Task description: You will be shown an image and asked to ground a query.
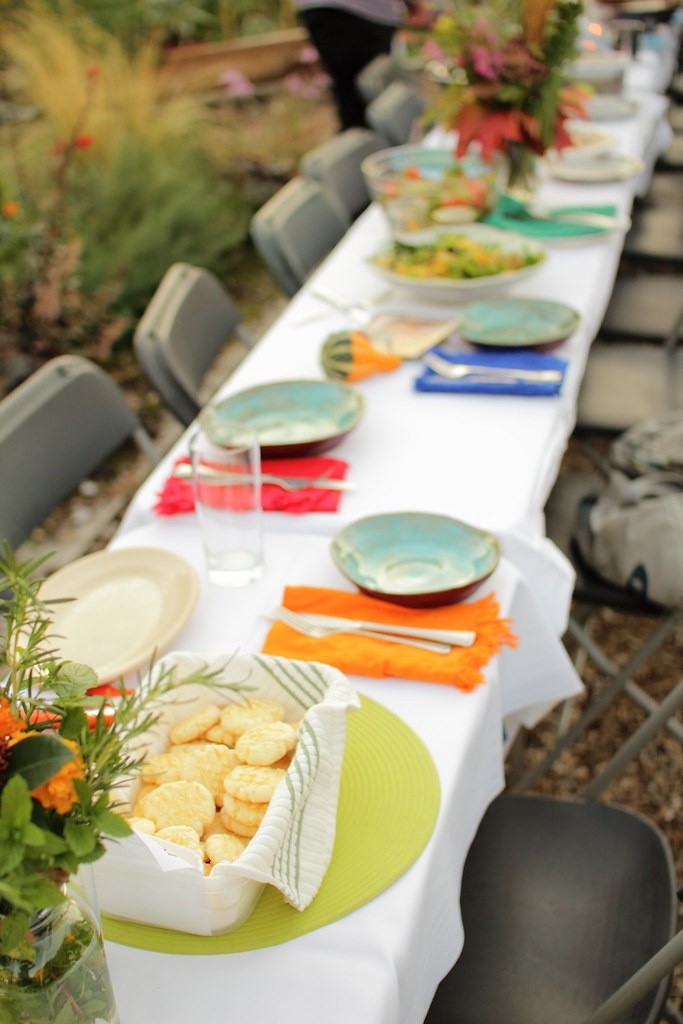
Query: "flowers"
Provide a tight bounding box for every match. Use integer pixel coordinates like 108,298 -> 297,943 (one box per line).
2,536 -> 265,989
396,1 -> 592,188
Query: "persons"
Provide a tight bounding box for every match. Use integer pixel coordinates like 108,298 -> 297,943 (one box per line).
296,1 -> 431,132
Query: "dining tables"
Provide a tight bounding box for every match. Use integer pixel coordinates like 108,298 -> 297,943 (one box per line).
1,1 -> 682,1021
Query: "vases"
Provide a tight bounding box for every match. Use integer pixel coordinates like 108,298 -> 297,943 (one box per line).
485,137 -> 550,203
1,884 -> 122,1024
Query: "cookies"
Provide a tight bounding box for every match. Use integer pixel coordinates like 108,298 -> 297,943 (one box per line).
127,694 -> 302,876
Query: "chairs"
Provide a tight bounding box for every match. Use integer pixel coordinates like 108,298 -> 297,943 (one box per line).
599,0 -> 683,347
576,302 -> 682,444
417,791 -> 683,1022
526,466 -> 683,809
302,127 -> 382,218
355,28 -> 424,149
248,175 -> 350,299
133,261 -> 256,424
1,353 -> 169,583
